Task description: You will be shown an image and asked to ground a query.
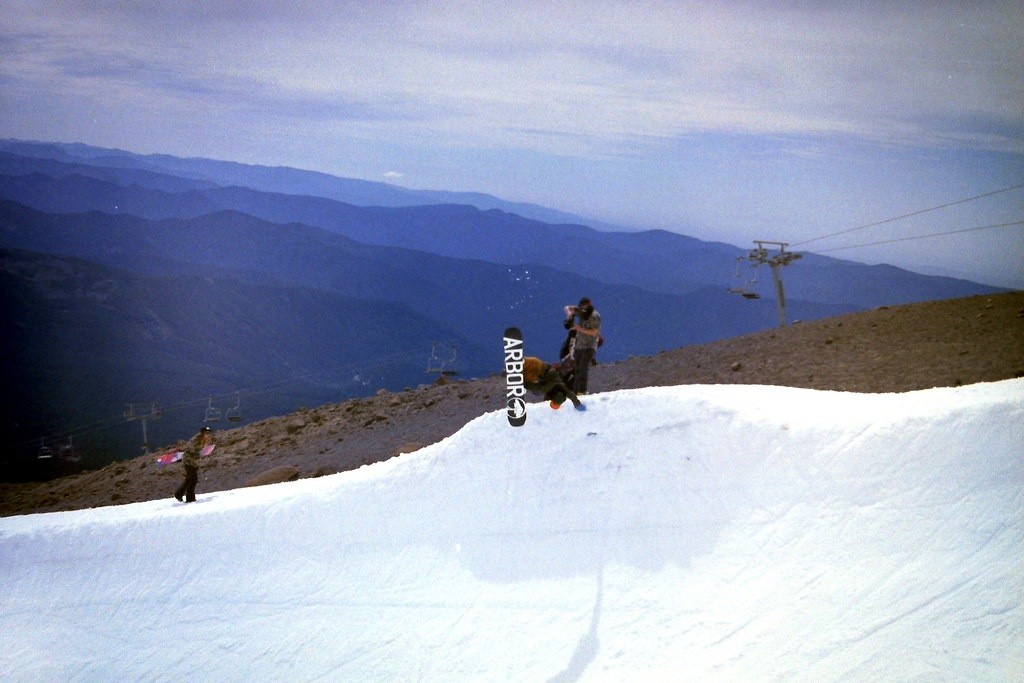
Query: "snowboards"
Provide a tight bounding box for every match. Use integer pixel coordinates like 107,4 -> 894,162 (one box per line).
503,327 -> 527,428
156,444 -> 215,465
560,313 -> 580,359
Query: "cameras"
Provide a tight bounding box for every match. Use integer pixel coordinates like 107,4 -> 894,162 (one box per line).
563,317 -> 574,329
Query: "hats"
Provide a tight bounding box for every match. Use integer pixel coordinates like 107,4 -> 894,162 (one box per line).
201,426 -> 212,432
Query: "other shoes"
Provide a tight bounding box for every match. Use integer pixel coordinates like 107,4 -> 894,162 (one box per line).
174,492 -> 185,502
186,498 -> 196,502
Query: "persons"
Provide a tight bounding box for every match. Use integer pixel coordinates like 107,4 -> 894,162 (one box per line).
501,297 -> 603,411
174,426 -> 213,503
